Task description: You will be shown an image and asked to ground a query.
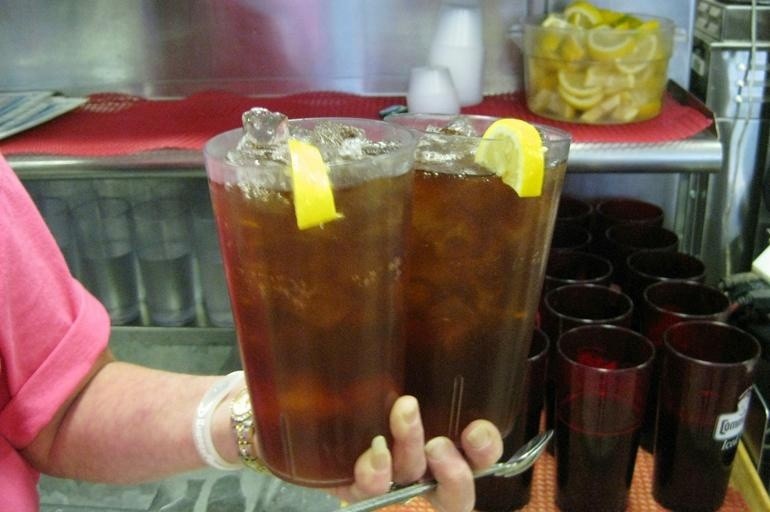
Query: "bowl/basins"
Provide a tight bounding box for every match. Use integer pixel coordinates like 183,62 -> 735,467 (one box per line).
508,10 -> 676,122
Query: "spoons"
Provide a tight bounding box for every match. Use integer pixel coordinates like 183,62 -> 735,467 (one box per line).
332,429 -> 554,510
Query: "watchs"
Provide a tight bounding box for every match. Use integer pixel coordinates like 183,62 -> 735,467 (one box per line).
231,386 -> 270,472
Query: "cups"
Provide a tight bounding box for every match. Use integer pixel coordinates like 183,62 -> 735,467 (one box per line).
621,251 -> 706,332
651,322 -> 761,511
203,115 -> 417,489
540,193 -> 683,289
37,193 -> 238,328
383,111 -> 551,452
542,284 -> 636,413
551,322 -> 652,511
641,284 -> 734,427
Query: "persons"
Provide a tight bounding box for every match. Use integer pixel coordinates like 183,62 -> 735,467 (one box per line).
0,153 -> 505,511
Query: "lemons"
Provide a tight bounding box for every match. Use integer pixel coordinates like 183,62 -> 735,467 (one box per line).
287,137 -> 345,231
528,1 -> 671,122
474,117 -> 546,198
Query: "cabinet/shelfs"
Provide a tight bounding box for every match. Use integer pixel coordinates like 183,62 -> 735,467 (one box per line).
2,76 -> 769,511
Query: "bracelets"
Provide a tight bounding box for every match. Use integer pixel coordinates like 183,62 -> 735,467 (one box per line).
193,370 -> 245,473
